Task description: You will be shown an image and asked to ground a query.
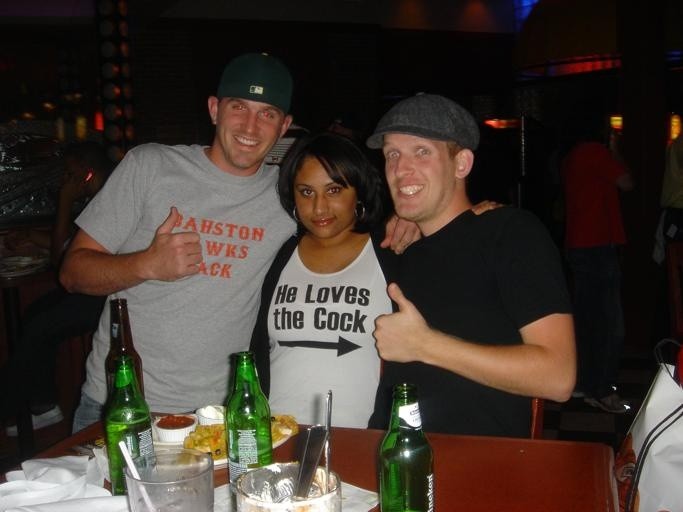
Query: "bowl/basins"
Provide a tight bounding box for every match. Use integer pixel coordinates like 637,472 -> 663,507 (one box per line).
195,405 -> 227,427
152,414 -> 198,445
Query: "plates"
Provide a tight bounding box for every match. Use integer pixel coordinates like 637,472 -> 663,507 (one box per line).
127,414 -> 291,478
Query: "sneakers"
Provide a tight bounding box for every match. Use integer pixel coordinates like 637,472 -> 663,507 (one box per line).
572,387 -> 631,416
5,403 -> 63,438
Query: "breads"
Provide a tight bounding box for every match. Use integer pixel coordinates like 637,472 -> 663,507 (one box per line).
174,412 -> 299,466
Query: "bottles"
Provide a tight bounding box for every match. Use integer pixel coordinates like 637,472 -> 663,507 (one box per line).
105,298 -> 145,405
101,352 -> 155,496
225,351 -> 273,498
378,380 -> 434,512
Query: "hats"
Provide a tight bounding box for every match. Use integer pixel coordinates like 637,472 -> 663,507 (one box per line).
217,52 -> 294,116
365,92 -> 481,150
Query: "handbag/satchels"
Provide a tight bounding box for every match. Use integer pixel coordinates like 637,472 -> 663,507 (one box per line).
610,339 -> 683,512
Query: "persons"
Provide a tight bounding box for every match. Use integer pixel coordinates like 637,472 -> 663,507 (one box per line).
0,139 -> 124,439
51,44 -> 426,440
252,123 -> 510,433
366,90 -> 580,443
658,132 -> 682,342
550,118 -> 635,415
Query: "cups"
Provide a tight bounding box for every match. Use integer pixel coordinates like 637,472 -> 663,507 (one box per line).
233,461 -> 342,512
121,447 -> 215,512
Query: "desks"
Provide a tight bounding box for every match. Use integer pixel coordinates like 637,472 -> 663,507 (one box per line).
0,411 -> 618,512
